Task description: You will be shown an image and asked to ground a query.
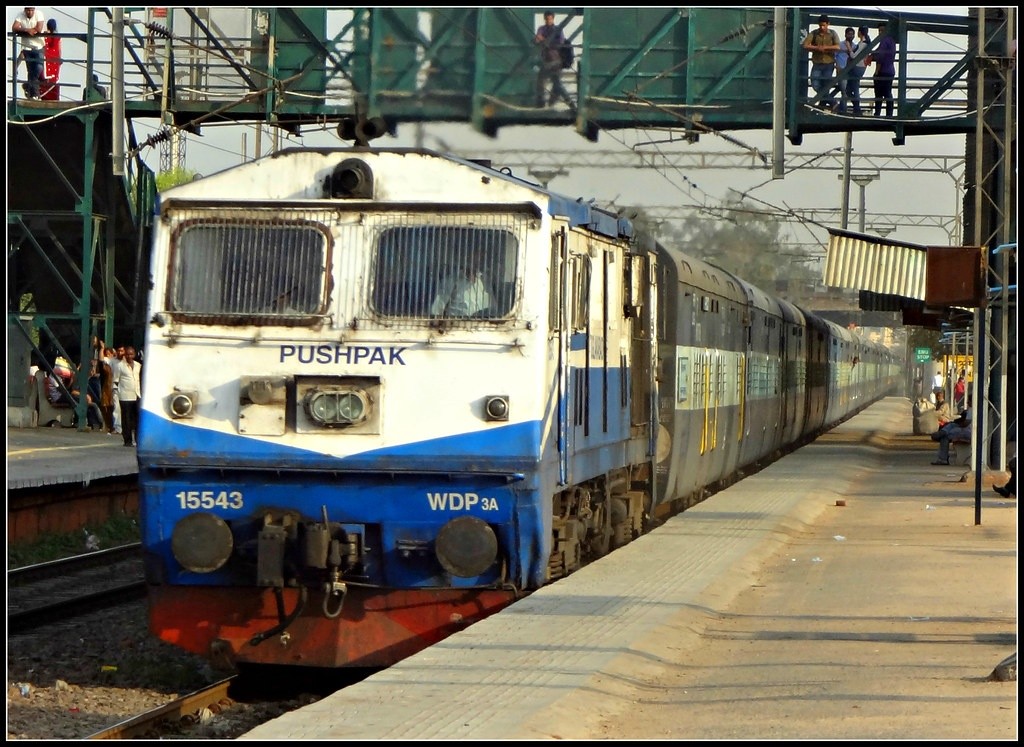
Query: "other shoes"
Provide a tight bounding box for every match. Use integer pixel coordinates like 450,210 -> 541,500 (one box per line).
931,432 -> 941,442
122,442 -> 135,447
992,484 -> 1010,498
930,460 -> 950,465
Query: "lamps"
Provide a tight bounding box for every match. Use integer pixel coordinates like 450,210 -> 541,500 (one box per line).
305,385 -> 374,427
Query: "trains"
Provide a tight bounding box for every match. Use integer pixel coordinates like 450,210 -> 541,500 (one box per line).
132,132 -> 911,669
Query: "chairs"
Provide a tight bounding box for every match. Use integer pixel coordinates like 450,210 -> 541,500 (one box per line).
35,370 -> 74,427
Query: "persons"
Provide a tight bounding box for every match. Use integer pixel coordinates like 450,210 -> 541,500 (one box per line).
532,11 -> 575,108
931,371 -> 1018,498
11,7 -> 107,102
29,335 -> 143,447
428,257 -> 499,318
261,282 -> 302,314
802,15 -> 896,117
